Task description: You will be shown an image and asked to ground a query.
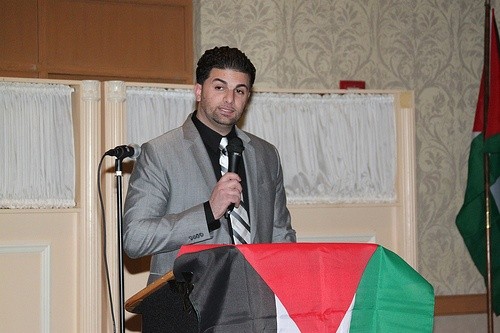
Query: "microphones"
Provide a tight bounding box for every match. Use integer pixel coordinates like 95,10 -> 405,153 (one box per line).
105,142 -> 142,159
227,137 -> 244,213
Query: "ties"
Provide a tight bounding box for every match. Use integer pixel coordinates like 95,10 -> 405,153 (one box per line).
218,137 -> 251,245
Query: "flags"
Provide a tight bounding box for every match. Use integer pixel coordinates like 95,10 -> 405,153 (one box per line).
172,243 -> 435,333
455,0 -> 500,320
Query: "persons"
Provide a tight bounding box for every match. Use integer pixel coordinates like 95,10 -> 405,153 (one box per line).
121,44 -> 297,333
